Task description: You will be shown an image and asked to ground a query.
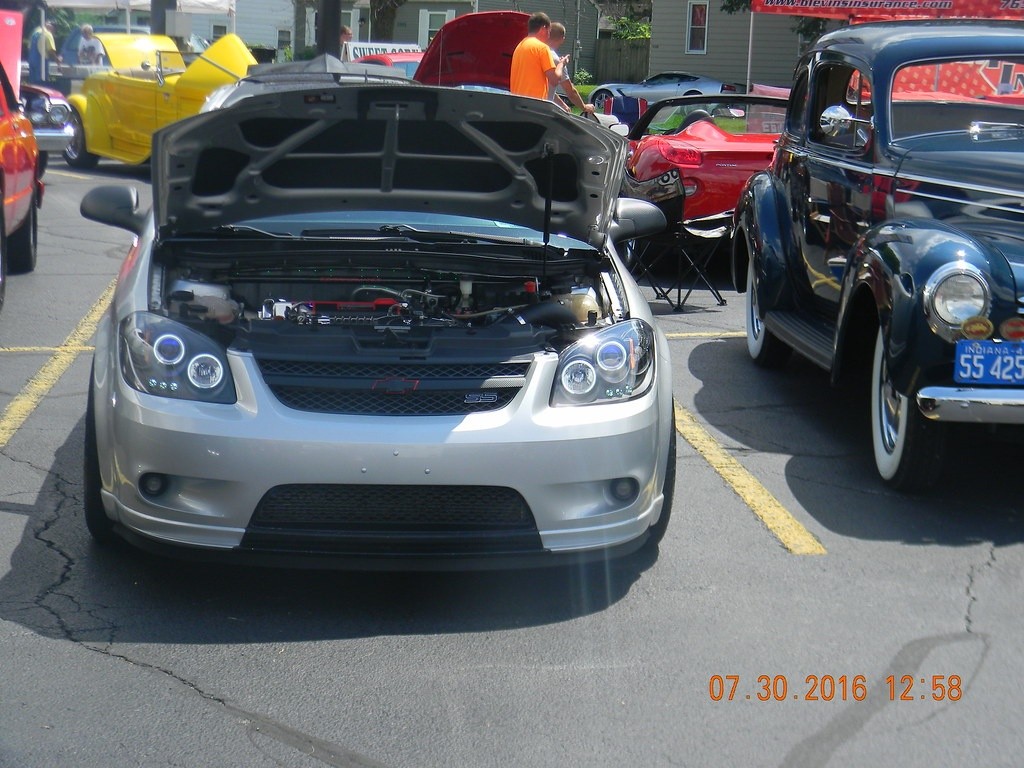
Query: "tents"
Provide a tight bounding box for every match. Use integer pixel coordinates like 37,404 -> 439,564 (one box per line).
744,0 -> 1024,133
36,0 -> 236,81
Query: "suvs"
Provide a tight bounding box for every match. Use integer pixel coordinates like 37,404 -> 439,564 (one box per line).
734,27 -> 1022,492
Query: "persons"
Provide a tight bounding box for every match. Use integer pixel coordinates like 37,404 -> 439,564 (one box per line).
27,21 -> 63,86
548,22 -> 595,115
329,26 -> 353,60
77,24 -> 105,66
510,12 -> 570,100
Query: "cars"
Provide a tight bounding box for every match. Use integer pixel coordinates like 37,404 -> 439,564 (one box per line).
82,75 -> 678,571
351,10 -> 532,92
0,11 -> 48,308
588,70 -> 736,128
19,85 -> 75,182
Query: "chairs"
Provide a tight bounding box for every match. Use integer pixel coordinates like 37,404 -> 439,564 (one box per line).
675,110 -> 718,136
622,171 -> 734,311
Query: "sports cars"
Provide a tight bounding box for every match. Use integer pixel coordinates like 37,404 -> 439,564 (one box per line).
65,31 -> 262,178
605,92 -> 802,259
